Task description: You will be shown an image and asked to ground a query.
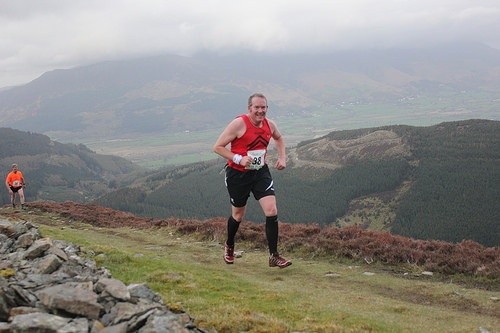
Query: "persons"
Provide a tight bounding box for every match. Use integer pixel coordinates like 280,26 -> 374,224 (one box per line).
214,94 -> 292,268
6,164 -> 27,210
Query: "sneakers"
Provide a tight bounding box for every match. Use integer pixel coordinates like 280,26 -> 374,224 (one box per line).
269,252 -> 292,268
224,240 -> 235,263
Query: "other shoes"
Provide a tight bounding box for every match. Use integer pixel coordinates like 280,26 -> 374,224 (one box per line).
21,204 -> 24,209
12,204 -> 17,209
